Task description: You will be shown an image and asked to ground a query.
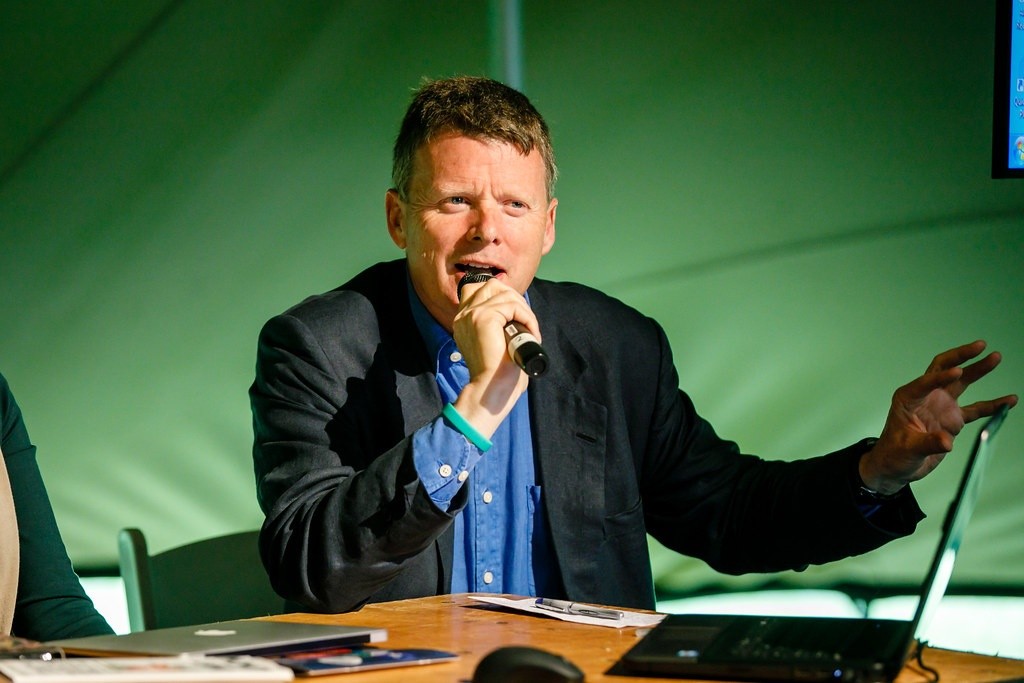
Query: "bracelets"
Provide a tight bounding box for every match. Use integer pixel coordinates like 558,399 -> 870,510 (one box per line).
857,441 -> 903,506
442,401 -> 493,453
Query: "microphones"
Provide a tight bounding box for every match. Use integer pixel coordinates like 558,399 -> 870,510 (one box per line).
457,268 -> 550,378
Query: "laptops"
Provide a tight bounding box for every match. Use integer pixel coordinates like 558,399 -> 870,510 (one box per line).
603,401 -> 1010,683
41,619 -> 389,660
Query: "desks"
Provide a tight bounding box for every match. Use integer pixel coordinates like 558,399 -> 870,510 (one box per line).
0,593 -> 1024,683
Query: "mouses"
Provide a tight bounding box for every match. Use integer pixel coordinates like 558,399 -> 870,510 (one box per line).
472,646 -> 583,683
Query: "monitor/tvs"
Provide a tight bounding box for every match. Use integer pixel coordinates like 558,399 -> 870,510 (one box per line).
990,0 -> 1024,179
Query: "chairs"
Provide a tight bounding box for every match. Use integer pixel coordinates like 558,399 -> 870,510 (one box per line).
117,528 -> 285,634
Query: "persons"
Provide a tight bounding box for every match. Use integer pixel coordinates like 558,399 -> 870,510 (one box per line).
0,373 -> 118,643
248,76 -> 1019,616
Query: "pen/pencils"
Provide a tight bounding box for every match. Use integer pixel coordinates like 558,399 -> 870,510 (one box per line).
534,596 -> 624,621
